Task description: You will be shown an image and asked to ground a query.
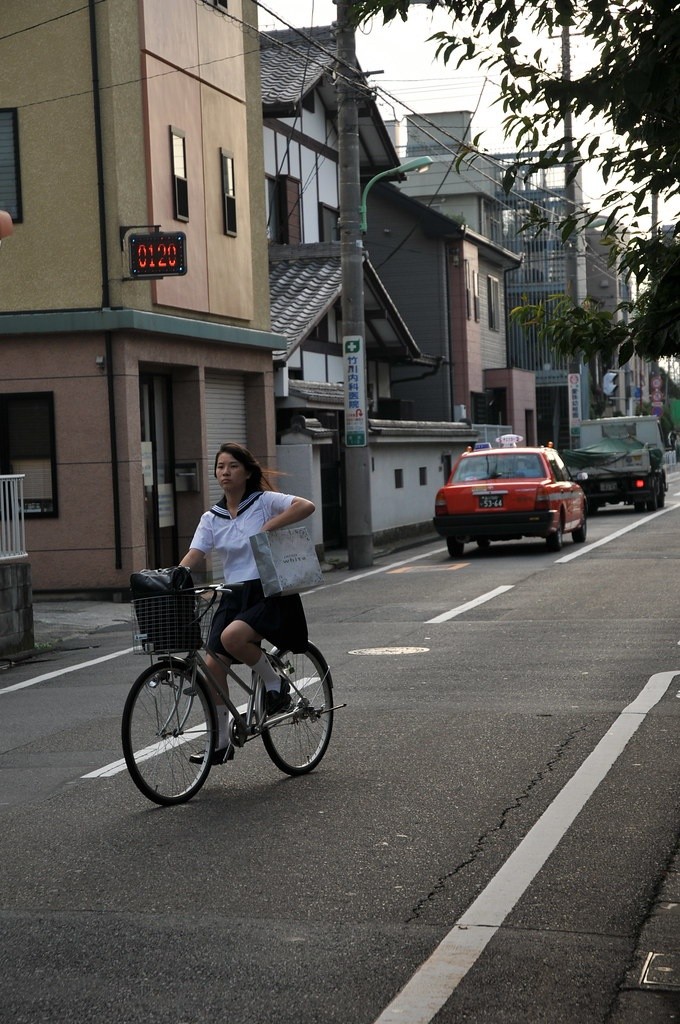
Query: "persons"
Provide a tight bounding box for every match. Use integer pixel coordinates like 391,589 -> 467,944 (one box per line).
178,441 -> 316,766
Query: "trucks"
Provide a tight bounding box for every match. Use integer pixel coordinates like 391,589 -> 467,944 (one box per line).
558,412 -> 676,515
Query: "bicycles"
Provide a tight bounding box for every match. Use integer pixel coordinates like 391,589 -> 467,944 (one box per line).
120,580 -> 349,807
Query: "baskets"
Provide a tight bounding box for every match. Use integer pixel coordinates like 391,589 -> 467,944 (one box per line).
131,594 -> 214,655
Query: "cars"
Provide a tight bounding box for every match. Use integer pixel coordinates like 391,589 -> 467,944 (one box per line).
433,434 -> 589,557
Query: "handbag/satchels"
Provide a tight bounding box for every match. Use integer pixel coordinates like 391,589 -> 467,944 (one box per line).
249,496 -> 324,597
129,566 -> 203,650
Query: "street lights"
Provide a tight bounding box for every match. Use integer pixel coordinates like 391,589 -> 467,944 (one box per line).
336,154 -> 435,570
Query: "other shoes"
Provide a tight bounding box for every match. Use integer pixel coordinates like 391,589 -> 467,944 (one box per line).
265,674 -> 286,715
190,746 -> 236,764
